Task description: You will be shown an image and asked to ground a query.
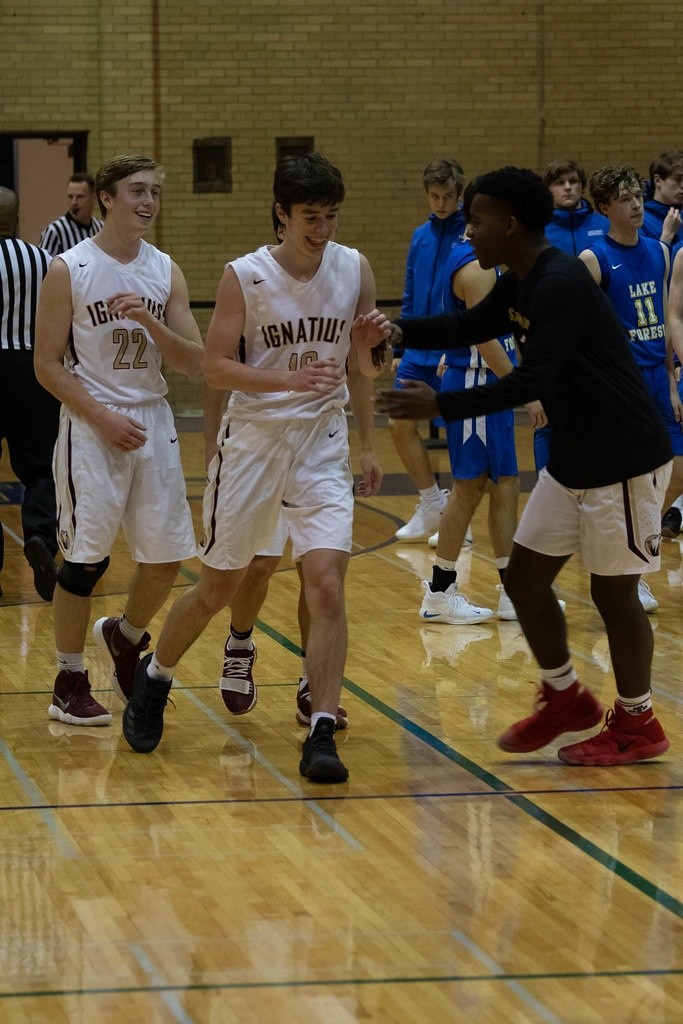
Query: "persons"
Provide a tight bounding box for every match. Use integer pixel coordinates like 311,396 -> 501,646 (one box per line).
220,198 -> 349,728
371,165 -> 673,767
39,173 -> 104,257
33,152 -> 204,728
576,165 -> 683,612
639,146 -> 683,245
419,183 -> 567,624
532,158 -> 610,479
389,157 -> 471,547
121,151 -> 392,785
661,245 -> 682,545
0,187 -> 57,602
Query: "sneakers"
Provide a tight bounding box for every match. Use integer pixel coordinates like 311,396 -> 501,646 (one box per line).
397,543 -> 473,582
496,676 -> 604,753
419,580 -> 492,624
660,507 -> 682,539
417,624 -> 494,666
93,615 -> 152,704
222,734 -> 257,805
219,634 -> 256,713
557,697 -> 670,765
300,717 -> 349,784
428,520 -> 473,546
396,488 -> 450,540
496,584 -> 567,621
49,720 -> 123,774
23,536 -> 59,602
48,669 -> 113,726
495,625 -> 535,665
637,579 -> 659,612
295,677 -> 348,729
121,652 -> 172,753
